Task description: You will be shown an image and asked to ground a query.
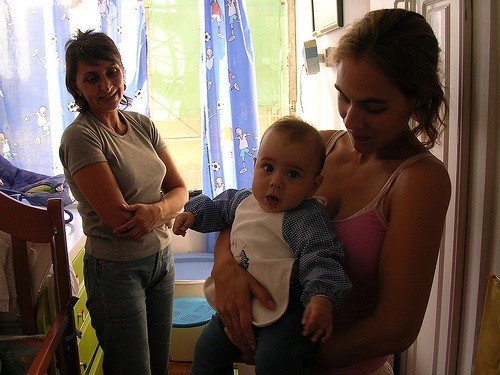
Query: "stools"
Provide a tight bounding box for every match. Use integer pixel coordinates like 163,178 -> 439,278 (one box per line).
169,297 -> 217,362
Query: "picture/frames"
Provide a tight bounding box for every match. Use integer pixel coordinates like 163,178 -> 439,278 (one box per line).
310,0 -> 343,37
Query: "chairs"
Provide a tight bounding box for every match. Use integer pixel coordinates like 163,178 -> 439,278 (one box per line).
0,190 -> 79,375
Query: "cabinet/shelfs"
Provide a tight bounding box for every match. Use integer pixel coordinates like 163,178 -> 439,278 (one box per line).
0,247 -> 103,375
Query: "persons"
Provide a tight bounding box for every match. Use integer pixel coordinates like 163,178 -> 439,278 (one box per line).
59,28 -> 189,375
173,115 -> 352,375
210,8 -> 452,375
